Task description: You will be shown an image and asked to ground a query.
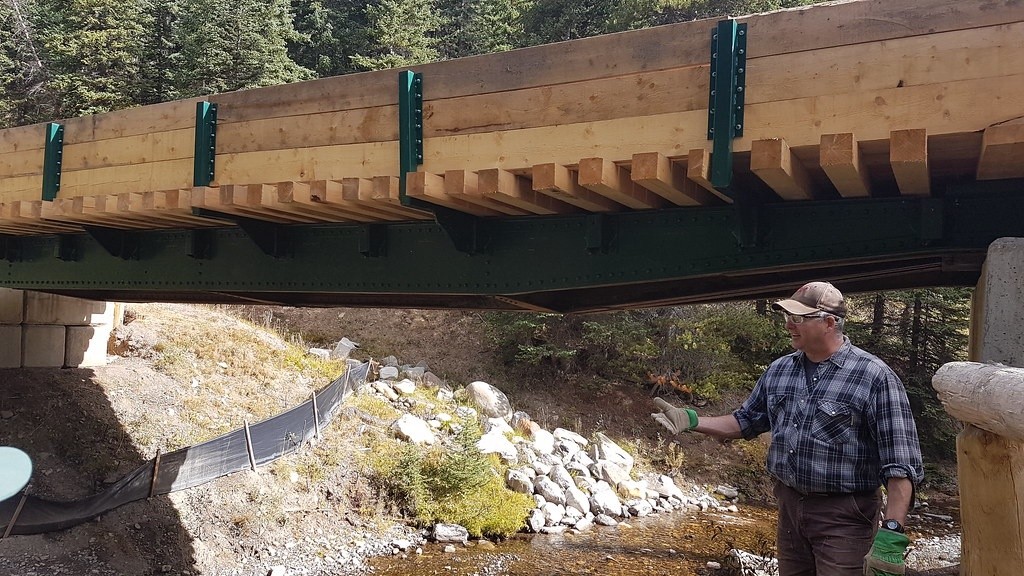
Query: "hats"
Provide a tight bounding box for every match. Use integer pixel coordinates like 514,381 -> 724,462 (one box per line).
772,281 -> 846,319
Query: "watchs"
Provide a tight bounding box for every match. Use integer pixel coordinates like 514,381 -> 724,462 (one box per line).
881,518 -> 904,533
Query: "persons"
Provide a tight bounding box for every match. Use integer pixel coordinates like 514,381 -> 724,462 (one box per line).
652,280 -> 924,576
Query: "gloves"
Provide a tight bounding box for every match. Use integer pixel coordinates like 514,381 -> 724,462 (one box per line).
650,397 -> 699,435
863,528 -> 911,576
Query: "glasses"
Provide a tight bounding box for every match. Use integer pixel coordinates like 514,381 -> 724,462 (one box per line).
783,311 -> 839,324
